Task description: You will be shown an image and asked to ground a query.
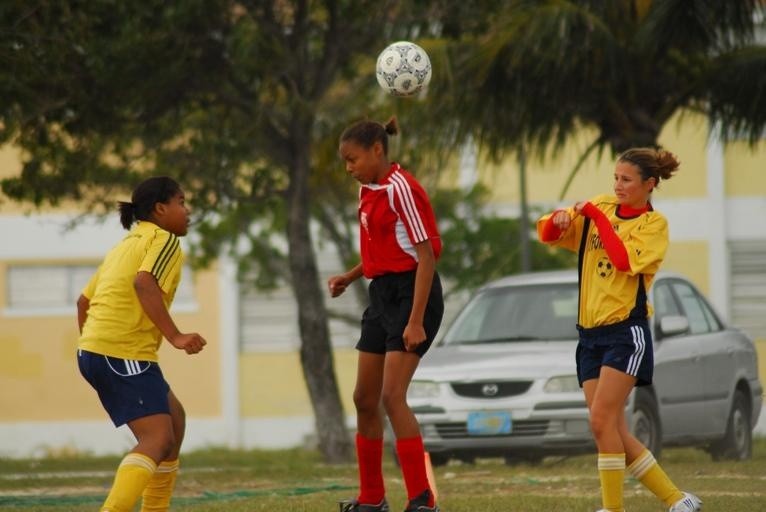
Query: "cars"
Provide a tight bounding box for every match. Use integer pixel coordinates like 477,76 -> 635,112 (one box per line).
404,271 -> 765,460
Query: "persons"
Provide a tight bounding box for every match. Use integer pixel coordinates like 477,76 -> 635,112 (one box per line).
76,176 -> 207,512
327,117 -> 444,512
537,146 -> 702,512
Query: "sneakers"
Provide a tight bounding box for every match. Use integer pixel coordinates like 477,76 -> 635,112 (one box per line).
333,493 -> 391,512
668,488 -> 704,512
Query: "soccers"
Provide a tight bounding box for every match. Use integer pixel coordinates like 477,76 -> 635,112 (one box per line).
375,40 -> 431,96
